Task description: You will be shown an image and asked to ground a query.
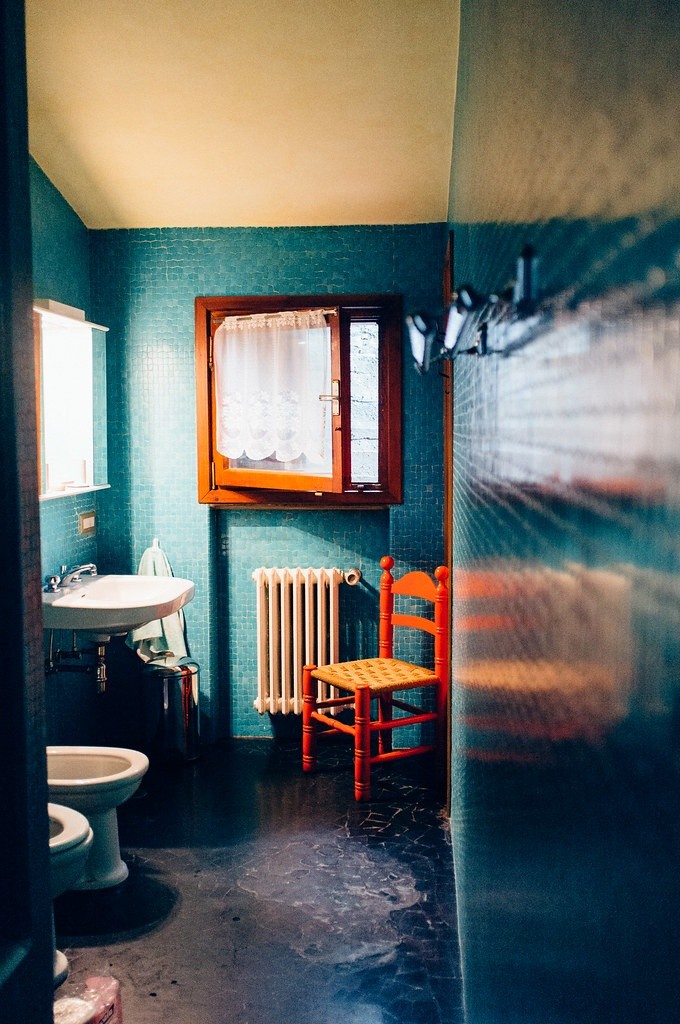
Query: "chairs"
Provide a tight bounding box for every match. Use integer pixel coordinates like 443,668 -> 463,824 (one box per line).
302,555 -> 452,800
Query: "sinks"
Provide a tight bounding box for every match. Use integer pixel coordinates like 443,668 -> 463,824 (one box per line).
42,574 -> 195,629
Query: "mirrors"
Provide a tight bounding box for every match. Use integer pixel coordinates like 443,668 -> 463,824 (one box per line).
33,297 -> 112,501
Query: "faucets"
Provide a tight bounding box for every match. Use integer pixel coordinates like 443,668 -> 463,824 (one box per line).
44,563 -> 98,593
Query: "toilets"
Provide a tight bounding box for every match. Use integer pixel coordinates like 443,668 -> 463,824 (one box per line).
47,803 -> 94,987
47,745 -> 149,892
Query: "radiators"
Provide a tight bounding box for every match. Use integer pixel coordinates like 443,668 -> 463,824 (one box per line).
251,569 -> 356,714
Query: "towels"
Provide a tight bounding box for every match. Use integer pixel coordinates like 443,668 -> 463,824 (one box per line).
125,536 -> 189,662
